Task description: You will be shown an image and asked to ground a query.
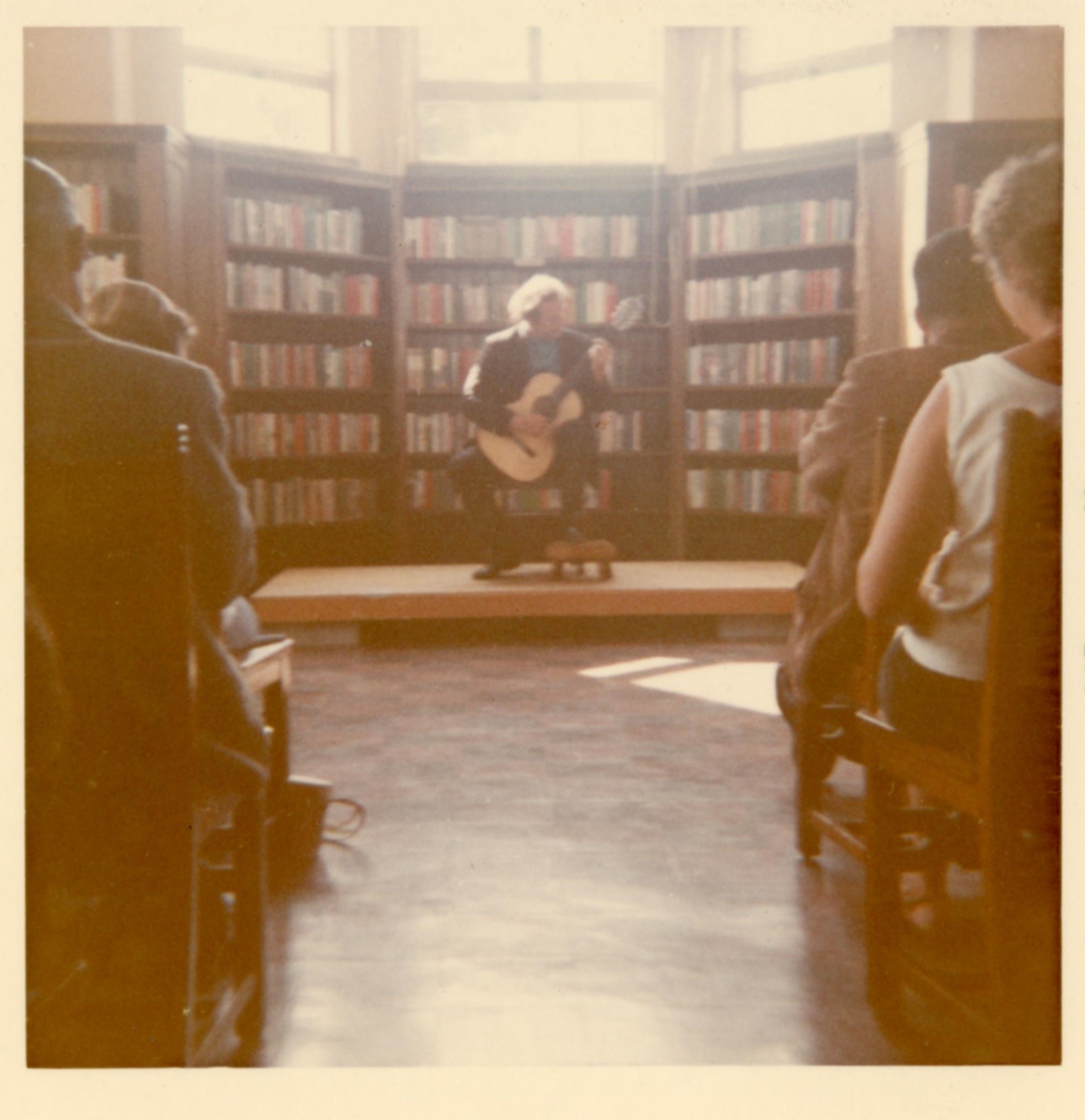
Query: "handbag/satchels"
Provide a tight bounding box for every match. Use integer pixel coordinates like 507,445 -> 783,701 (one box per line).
282,773 -> 364,860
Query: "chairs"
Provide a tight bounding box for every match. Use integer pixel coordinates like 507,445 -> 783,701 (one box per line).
795,406 -> 1061,1064
29,426 -> 295,1067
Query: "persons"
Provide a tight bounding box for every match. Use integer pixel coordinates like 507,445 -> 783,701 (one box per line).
23,152 -> 276,992
81,277 -> 260,653
786,224 -> 1028,712
855,138 -> 1064,763
445,271 -> 617,581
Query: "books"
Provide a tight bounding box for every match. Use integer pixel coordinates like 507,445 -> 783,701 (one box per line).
685,469 -> 800,516
226,196 -> 364,255
407,466 -> 610,512
685,266 -> 840,321
70,247 -> 126,326
686,335 -> 839,387
225,339 -> 374,388
404,409 -> 642,452
68,183 -> 113,232
225,260 -> 380,316
684,408 -> 817,453
401,213 -> 640,259
406,347 -> 632,392
247,477 -> 363,526
231,412 -> 381,456
686,196 -> 852,259
409,280 -> 618,322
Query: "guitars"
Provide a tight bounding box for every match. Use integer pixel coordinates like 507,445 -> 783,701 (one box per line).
475,293 -> 653,484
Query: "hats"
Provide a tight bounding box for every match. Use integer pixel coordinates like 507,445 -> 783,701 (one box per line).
914,225 -> 990,308
91,281 -> 174,332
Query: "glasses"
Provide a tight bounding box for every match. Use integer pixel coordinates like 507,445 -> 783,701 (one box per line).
550,311 -> 563,320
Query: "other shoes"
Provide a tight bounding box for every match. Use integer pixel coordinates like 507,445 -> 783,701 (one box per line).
561,526 -> 584,545
472,565 -> 501,578
897,854 -> 945,936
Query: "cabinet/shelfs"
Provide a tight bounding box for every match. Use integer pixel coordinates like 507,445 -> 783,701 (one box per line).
177,137 -> 402,571
675,131 -> 897,559
898,116 -> 1068,354
391,157 -> 674,568
24,120 -> 185,314
